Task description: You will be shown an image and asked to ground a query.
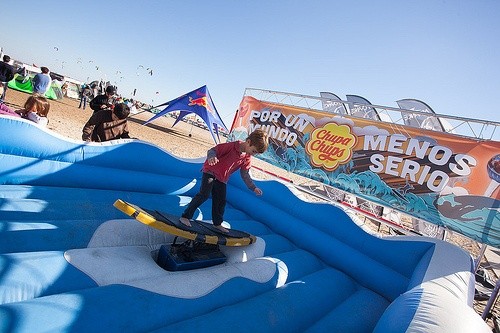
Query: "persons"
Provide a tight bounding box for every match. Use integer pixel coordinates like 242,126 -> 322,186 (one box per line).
179,128 -> 270,233
0,55 -> 131,142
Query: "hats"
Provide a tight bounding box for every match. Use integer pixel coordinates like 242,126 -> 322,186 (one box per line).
106,85 -> 116,92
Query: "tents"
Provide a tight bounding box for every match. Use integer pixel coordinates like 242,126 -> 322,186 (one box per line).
131,84 -> 230,145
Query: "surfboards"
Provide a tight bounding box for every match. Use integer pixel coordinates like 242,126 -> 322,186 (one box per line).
112,196 -> 256,248
